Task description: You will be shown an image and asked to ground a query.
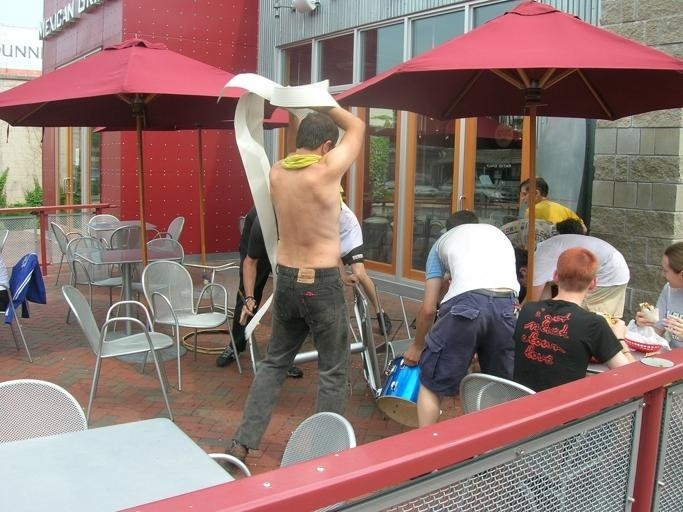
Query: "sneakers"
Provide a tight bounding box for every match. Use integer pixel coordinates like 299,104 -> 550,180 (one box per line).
225,439 -> 248,468
288,366 -> 303,378
216,345 -> 241,367
376,312 -> 392,335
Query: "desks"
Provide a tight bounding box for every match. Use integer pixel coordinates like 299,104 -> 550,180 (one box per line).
89,220 -> 155,287
1,418 -> 235,511
247,283 -> 380,402
74,250 -> 187,362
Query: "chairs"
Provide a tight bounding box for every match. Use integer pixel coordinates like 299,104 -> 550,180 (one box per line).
346,292 -> 413,382
208,412 -> 356,482
61,284 -> 177,423
114,238 -> 184,331
183,261 -> 240,311
149,216 -> 185,240
0,253 -> 38,363
88,214 -> 120,248
1,379 -> 87,444
0,230 -> 9,249
457,374 -> 608,512
67,236 -> 124,331
141,260 -> 242,391
50,222 -> 88,286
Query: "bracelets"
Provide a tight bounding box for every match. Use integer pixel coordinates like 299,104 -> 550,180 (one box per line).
617,338 -> 625,341
245,296 -> 255,303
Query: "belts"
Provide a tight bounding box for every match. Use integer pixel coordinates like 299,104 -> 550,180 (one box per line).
473,288 -> 516,299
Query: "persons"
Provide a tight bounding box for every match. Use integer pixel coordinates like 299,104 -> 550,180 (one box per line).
220,105 -> 367,476
0,253 -> 12,311
515,233 -> 631,320
216,207 -> 304,378
635,242 -> 683,348
513,247 -> 636,392
403,210 -> 522,428
338,201 -> 392,335
519,177 -> 588,234
499,217 -> 585,250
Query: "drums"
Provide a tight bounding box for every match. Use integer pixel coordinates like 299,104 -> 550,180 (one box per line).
375,352 -> 421,427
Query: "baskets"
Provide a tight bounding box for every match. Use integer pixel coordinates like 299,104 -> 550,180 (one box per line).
624,337 -> 663,353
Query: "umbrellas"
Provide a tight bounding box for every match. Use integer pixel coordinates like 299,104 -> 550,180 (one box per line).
370,111 -> 522,187
331,0 -> 683,302
92,105 -> 291,265
0,39 -> 268,268
